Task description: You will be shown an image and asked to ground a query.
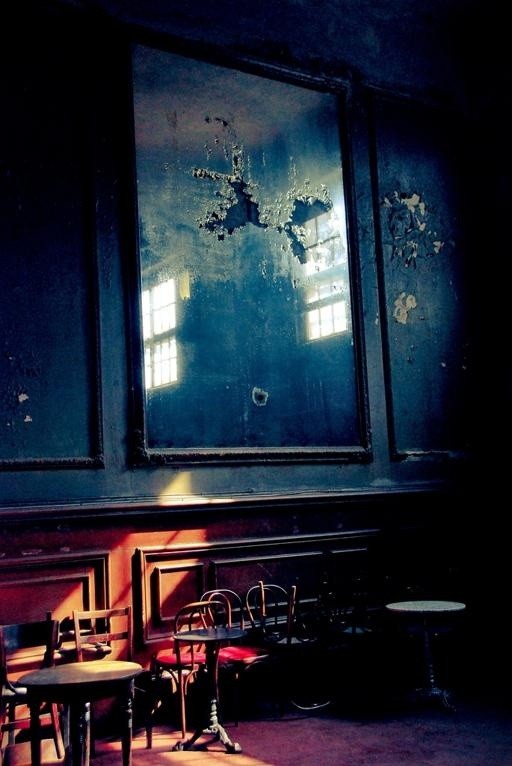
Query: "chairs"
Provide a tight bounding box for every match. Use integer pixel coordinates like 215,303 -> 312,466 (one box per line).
0,618 -> 66,766
158,599 -> 224,738
199,586 -> 271,726
73,604 -> 133,757
246,584 -> 319,715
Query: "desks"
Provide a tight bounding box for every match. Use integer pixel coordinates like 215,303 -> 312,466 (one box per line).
385,601 -> 467,706
172,631 -> 249,754
16,659 -> 142,766
